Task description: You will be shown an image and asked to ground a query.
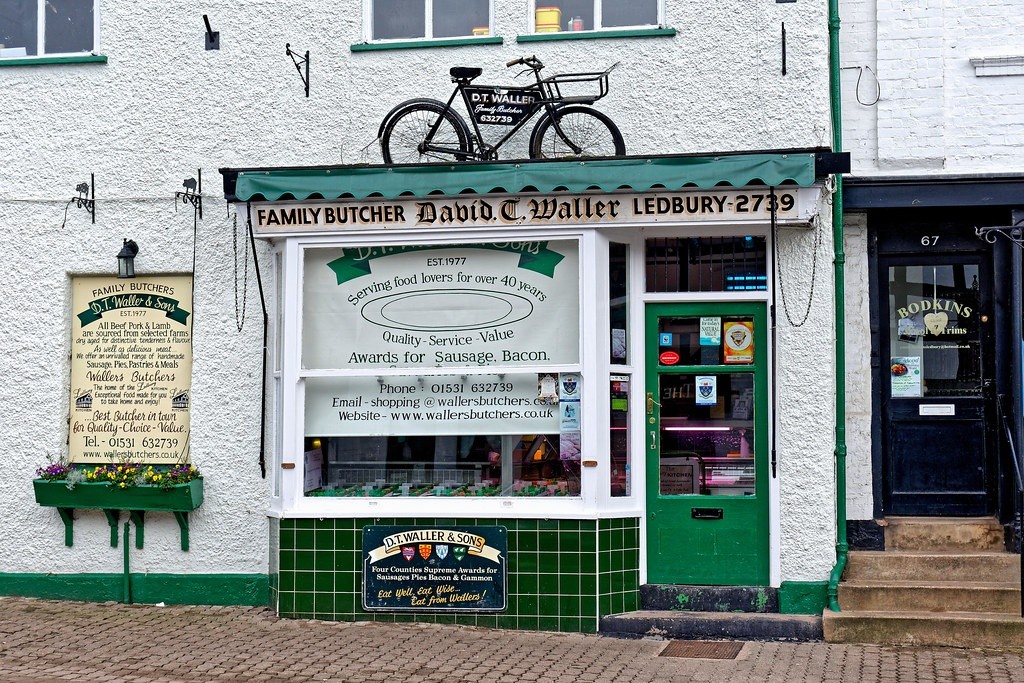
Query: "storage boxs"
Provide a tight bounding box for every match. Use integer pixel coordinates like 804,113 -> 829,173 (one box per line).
535,26 -> 563,32
472,26 -> 489,36
535,6 -> 563,26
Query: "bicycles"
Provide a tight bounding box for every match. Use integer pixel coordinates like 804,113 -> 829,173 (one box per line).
381,54 -> 626,167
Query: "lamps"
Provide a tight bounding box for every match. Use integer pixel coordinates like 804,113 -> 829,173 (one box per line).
116,238 -> 140,279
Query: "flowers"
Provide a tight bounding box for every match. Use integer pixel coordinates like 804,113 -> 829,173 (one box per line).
35,451 -> 204,492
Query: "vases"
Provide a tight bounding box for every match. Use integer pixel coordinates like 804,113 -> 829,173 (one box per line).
32,465 -> 204,552
